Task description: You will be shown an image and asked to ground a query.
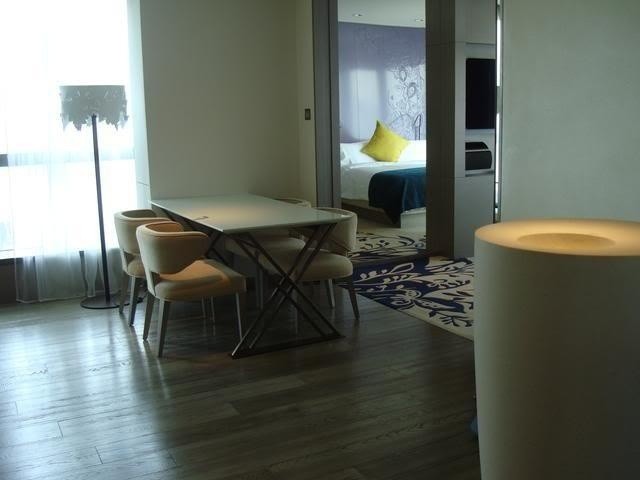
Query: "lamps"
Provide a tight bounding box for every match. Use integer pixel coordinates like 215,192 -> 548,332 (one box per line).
58,83 -> 144,312
469,216 -> 639,479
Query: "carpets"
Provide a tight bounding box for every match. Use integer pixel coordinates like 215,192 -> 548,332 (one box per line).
327,213 -> 474,342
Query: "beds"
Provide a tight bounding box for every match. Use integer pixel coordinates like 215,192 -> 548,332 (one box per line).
339,139 -> 427,230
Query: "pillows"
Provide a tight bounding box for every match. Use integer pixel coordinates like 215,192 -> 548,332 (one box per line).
359,119 -> 410,164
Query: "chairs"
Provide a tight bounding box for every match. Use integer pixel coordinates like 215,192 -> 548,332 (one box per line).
256,202 -> 362,337
223,197 -> 317,312
135,216 -> 247,359
113,208 -> 211,328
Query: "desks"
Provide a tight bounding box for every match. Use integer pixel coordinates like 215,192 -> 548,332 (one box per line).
150,191 -> 354,360
